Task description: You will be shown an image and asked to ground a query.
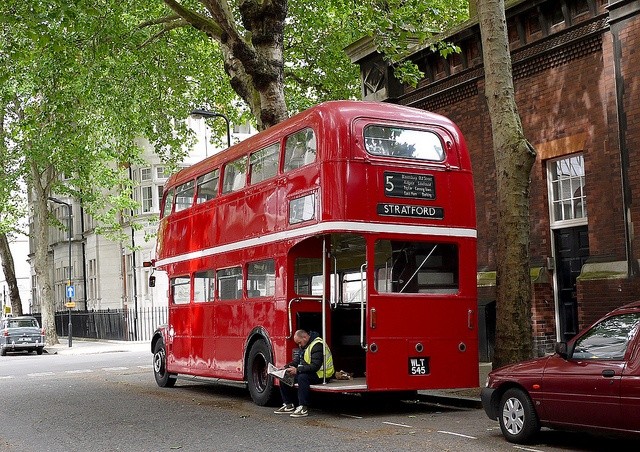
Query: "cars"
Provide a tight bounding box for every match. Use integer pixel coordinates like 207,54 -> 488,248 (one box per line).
481,300 -> 640,443
0,316 -> 45,356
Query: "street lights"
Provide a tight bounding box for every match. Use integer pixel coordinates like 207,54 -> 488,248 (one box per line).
188,109 -> 231,149
46,197 -> 75,347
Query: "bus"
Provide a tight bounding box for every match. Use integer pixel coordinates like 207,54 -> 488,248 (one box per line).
143,100 -> 480,406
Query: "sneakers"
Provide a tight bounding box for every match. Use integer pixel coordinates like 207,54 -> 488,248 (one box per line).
289,405 -> 308,417
273,403 -> 295,414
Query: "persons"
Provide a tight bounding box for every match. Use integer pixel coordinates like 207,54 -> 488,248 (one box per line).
273,330 -> 336,418
